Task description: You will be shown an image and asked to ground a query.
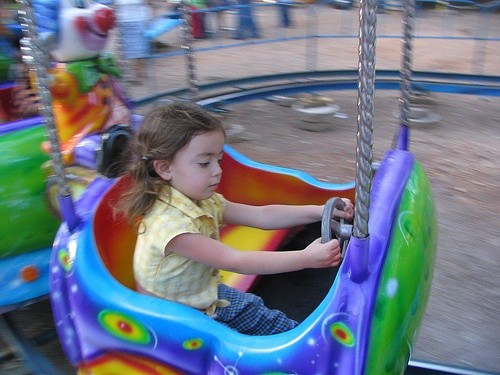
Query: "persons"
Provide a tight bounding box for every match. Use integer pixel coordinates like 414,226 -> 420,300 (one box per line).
104,100 -> 353,336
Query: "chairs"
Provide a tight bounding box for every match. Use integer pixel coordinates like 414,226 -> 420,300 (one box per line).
94,150 -> 303,334
